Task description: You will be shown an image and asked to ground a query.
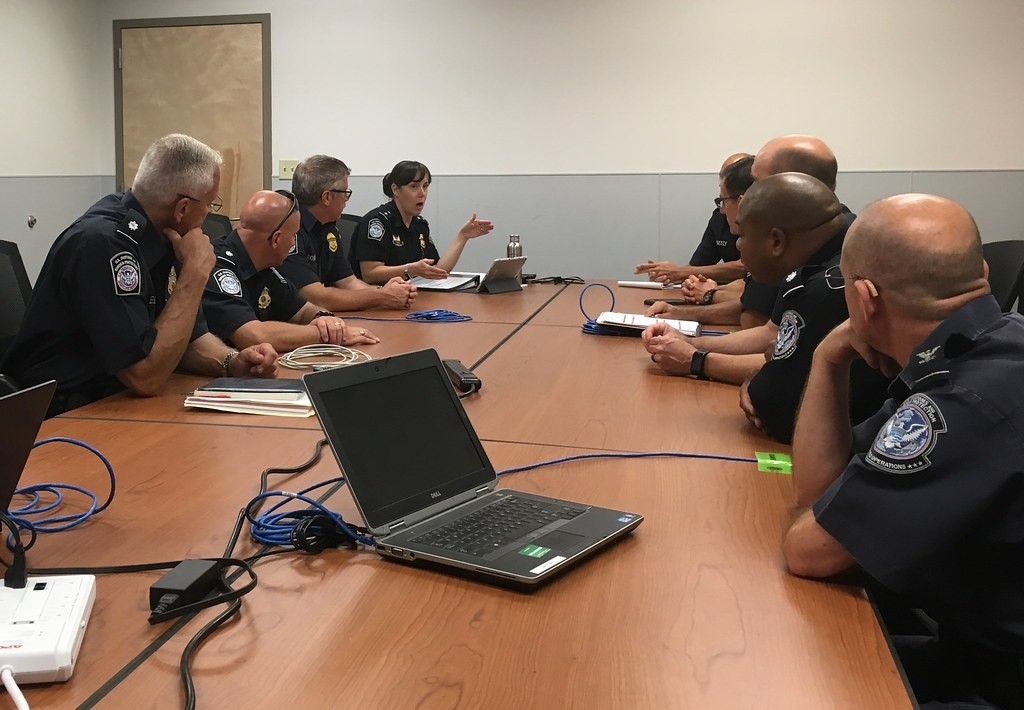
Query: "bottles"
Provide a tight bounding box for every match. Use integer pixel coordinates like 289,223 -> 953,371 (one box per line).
507,235 -> 523,285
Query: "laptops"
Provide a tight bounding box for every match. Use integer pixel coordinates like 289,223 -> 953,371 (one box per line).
300,348 -> 644,593
0,379 -> 59,521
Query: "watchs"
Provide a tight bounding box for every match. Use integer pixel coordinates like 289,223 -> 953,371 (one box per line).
703,288 -> 717,305
690,349 -> 714,381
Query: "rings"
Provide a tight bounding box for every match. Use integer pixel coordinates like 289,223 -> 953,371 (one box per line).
651,354 -> 657,362
480,227 -> 483,231
650,271 -> 655,278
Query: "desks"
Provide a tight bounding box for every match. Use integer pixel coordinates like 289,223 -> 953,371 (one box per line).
0,281 -> 914,710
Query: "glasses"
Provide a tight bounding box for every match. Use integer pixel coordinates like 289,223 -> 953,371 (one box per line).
320,188 -> 352,201
176,193 -> 223,212
714,194 -> 744,209
268,190 -> 299,240
824,265 -> 878,297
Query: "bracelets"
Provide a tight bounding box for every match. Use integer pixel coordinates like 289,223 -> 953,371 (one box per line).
222,351 -> 239,378
307,311 -> 335,324
404,263 -> 417,280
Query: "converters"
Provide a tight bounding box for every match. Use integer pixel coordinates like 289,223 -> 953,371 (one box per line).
149,560 -> 222,612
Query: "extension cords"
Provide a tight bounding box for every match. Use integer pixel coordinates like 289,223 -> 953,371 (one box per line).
0,574 -> 96,687
442,359 -> 482,393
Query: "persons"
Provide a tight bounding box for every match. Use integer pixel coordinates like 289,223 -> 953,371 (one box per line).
633,134 -> 857,385
201,188 -> 381,352
0,134 -> 280,420
347,161 -> 494,286
736,172 -> 903,445
272,154 -> 417,312
782,192 -> 1024,710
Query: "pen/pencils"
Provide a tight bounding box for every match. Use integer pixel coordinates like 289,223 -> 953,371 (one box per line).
198,395 -> 231,398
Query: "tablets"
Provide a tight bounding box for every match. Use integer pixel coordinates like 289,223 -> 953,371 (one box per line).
476,256 -> 529,290
198,376 -> 305,393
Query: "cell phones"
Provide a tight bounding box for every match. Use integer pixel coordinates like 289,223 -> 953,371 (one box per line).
644,298 -> 688,305
